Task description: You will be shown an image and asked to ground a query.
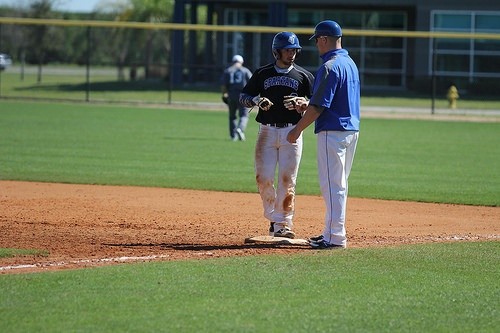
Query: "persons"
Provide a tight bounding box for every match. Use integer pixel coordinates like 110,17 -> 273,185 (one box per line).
239,31 -> 315,239
287,20 -> 361,248
221,55 -> 254,142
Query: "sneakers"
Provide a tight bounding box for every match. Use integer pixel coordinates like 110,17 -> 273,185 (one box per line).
309,240 -> 345,249
309,235 -> 324,242
269,221 -> 276,236
274,227 -> 295,238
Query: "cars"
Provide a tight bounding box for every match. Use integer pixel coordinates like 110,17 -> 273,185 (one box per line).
0,54 -> 12,70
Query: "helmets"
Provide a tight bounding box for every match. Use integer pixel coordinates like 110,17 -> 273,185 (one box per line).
232,55 -> 243,63
309,20 -> 342,40
272,32 -> 302,60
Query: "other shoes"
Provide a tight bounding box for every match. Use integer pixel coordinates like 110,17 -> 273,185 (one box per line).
237,129 -> 244,141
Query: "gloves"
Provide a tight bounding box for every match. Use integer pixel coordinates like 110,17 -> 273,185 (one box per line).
283,95 -> 306,110
251,95 -> 273,111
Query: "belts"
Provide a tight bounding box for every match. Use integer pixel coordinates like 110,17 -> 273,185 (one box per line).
263,123 -> 294,127
